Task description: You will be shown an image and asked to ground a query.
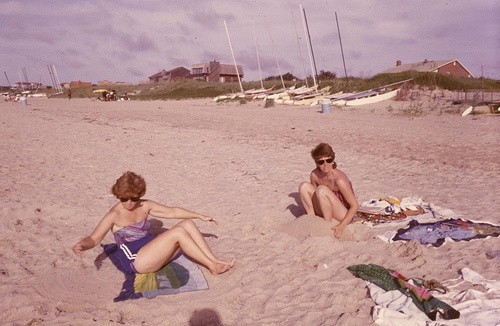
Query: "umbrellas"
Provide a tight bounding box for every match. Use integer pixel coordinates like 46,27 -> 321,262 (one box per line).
92,89 -> 109,96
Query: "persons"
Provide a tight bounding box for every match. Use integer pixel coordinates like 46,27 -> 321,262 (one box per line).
72,172 -> 237,276
102,90 -> 116,99
68,89 -> 72,101
298,143 -> 359,239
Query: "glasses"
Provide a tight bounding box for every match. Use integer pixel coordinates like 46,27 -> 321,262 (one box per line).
316,157 -> 334,165
119,197 -> 139,202
